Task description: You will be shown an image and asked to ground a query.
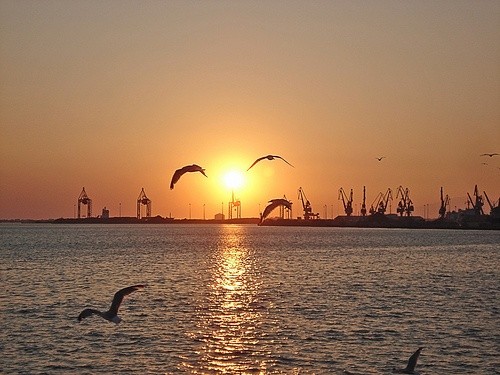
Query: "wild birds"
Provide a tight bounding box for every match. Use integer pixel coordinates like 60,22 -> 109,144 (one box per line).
246,155 -> 296,171
480,153 -> 500,157
169,164 -> 209,191
77,283 -> 147,325
376,157 -> 383,161
